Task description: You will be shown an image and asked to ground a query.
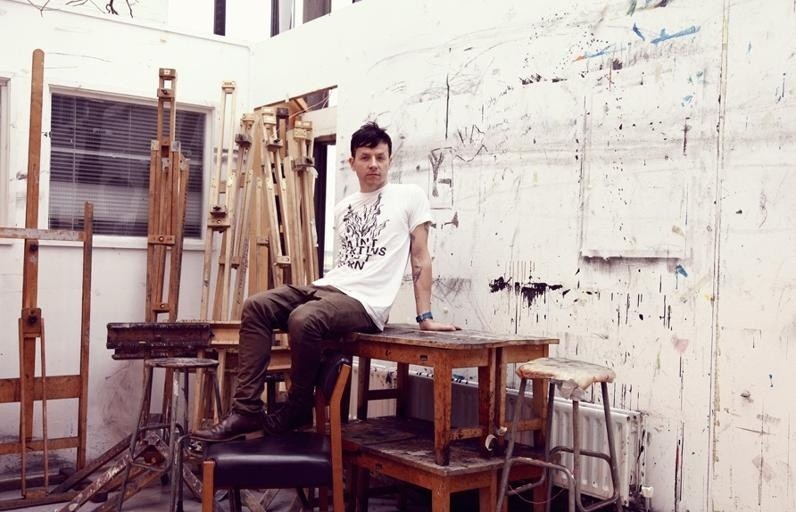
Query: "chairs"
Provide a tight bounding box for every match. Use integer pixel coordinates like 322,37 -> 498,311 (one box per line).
201,352 -> 351,512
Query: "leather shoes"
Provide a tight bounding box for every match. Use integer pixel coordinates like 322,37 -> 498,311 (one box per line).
189,411 -> 266,444
261,405 -> 314,434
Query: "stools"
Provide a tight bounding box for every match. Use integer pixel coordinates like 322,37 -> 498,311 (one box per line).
118,356 -> 224,512
493,356 -> 621,512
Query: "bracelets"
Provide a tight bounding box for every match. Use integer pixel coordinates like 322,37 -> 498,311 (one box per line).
414,311 -> 433,323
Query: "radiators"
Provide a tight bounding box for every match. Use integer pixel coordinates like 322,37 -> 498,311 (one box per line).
349,362 -> 641,509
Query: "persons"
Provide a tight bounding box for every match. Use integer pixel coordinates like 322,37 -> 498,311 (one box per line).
185,119 -> 461,444
